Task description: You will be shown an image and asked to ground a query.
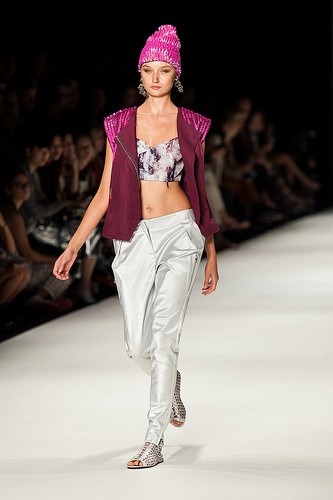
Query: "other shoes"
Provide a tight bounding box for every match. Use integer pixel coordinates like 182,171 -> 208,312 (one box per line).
126,439 -> 164,468
168,370 -> 185,428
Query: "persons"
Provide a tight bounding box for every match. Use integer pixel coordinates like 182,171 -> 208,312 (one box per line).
1,70 -> 333,342
52,23 -> 219,469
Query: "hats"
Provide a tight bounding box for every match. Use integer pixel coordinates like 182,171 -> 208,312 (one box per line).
137,25 -> 181,78
